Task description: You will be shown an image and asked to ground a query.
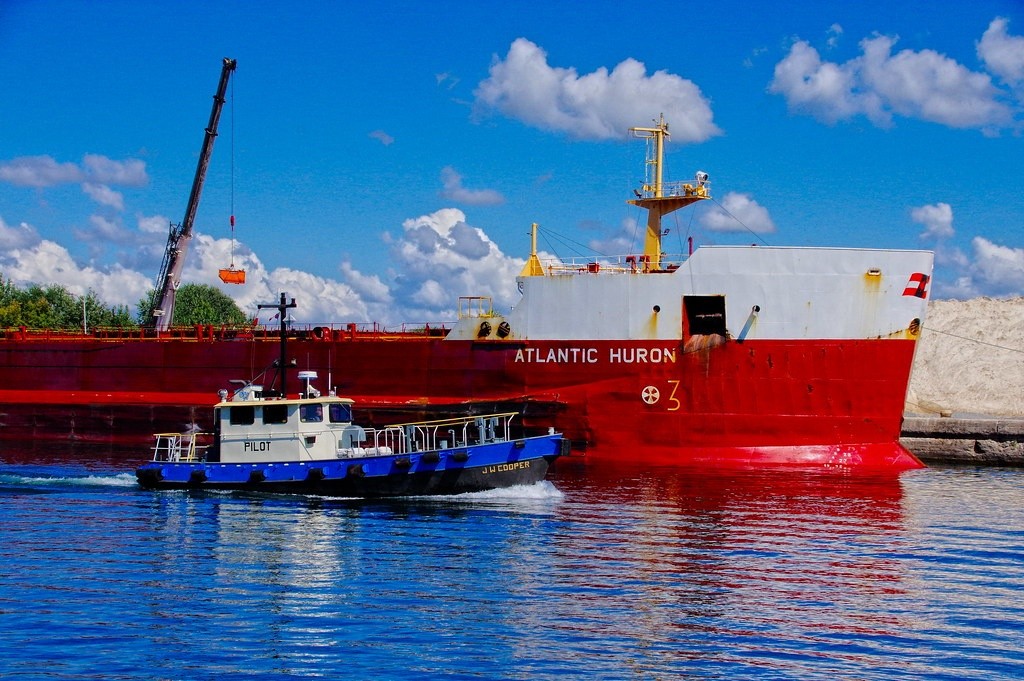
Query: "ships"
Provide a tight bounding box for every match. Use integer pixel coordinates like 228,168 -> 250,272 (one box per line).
0,114 -> 943,480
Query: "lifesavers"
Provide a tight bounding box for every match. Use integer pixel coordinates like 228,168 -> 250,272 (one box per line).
265,396 -> 282,400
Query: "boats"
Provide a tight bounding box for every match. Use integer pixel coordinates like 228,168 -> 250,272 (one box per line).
132,291 -> 572,500
